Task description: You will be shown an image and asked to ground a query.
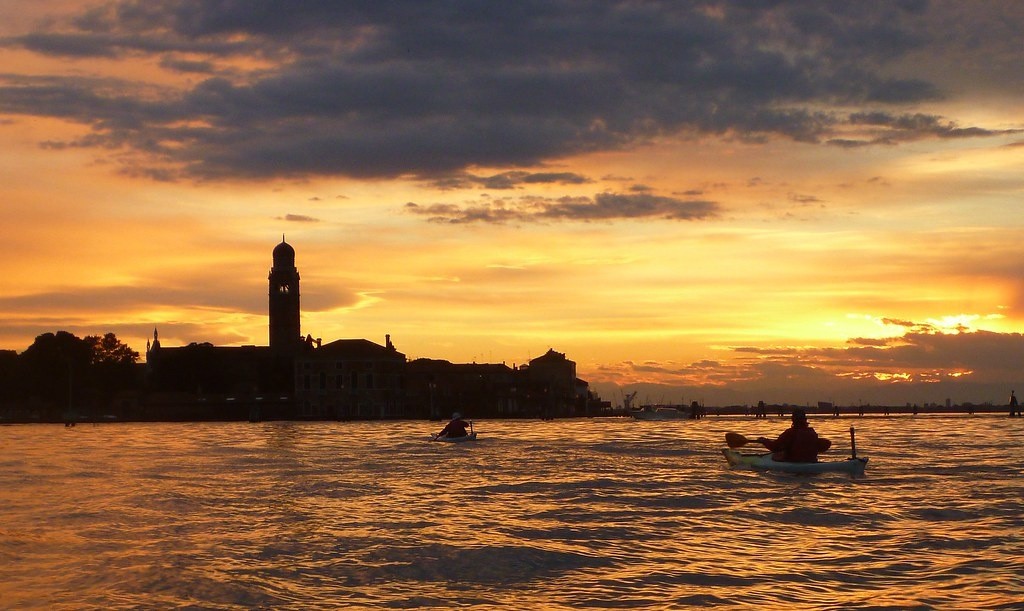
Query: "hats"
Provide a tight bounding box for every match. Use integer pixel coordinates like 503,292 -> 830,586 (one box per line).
452,412 -> 462,420
792,410 -> 808,419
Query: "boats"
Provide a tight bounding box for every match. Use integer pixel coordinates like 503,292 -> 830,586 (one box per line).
430,418 -> 478,442
719,424 -> 869,475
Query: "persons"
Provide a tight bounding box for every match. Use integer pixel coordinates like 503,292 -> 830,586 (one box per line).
758,410 -> 819,463
439,413 -> 469,438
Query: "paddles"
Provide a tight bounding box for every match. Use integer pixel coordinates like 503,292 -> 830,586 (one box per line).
725,432 -> 832,454
